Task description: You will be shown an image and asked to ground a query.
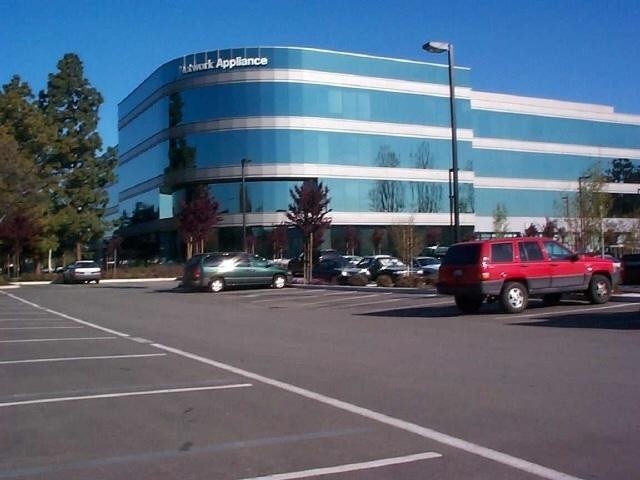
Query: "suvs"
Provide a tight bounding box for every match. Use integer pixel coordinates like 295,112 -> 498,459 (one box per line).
439,238 -> 626,314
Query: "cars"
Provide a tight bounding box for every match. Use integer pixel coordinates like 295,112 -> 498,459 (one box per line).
287,246 -> 449,287
62,260 -> 101,284
183,251 -> 292,293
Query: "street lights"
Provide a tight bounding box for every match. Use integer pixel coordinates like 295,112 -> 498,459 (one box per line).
242,159 -> 252,251
579,176 -> 591,237
422,40 -> 460,242
